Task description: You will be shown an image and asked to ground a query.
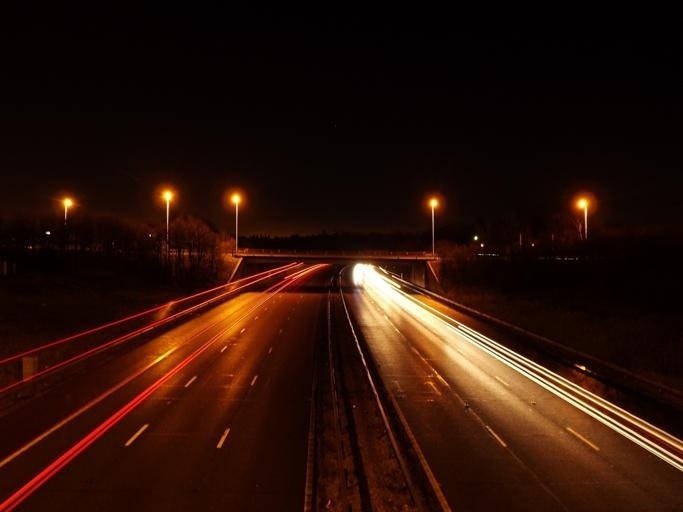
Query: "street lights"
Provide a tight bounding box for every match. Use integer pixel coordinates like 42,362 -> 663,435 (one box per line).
430,198 -> 437,255
164,191 -> 172,258
64,198 -> 71,224
578,199 -> 587,240
232,194 -> 239,252
